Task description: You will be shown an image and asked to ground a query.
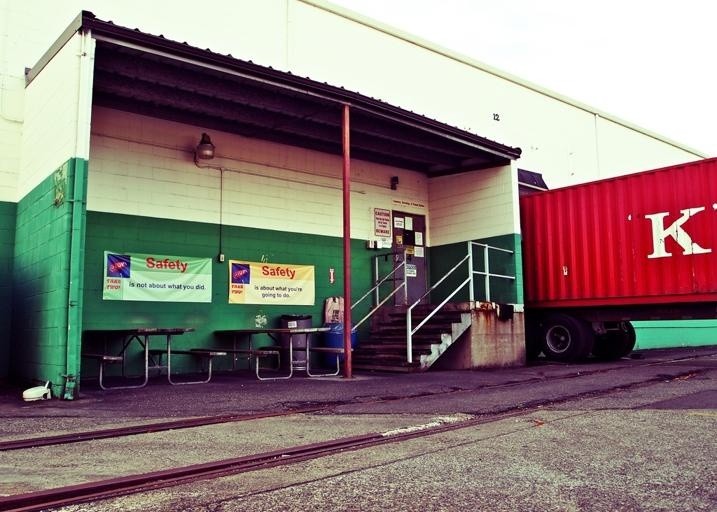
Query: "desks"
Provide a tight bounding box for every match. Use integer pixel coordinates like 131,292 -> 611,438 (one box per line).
213,327 -> 332,382
81,326 -> 196,391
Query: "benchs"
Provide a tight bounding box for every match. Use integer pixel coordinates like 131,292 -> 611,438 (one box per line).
82,346 -> 356,392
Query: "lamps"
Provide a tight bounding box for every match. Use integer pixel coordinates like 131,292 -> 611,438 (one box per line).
390,175 -> 400,190
193,131 -> 217,168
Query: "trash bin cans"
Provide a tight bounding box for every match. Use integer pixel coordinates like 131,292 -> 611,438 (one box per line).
320,323 -> 359,375
280,313 -> 312,373
